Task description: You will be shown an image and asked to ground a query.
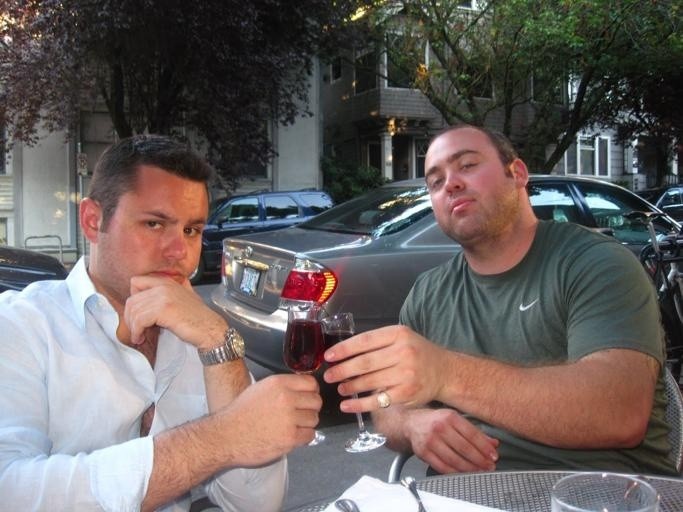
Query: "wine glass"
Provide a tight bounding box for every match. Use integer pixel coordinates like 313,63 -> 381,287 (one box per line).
283,302 -> 326,449
318,311 -> 389,453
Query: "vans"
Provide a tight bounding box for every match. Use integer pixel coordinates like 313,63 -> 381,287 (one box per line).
189,186 -> 336,287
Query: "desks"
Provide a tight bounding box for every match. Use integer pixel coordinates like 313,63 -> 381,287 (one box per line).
292,469 -> 683,512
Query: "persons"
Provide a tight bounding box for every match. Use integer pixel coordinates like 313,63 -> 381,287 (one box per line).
0,135 -> 323,512
323,124 -> 683,512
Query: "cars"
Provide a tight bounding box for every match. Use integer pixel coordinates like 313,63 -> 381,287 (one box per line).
594,184 -> 683,231
205,171 -> 681,385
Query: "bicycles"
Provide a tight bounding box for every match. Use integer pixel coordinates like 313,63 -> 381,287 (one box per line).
594,206 -> 682,329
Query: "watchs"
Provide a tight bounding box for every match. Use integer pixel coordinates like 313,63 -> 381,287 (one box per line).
198,326 -> 246,367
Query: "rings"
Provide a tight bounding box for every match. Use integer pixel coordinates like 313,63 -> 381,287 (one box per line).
378,392 -> 392,409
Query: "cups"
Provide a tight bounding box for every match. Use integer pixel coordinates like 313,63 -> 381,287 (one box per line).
549,472 -> 661,511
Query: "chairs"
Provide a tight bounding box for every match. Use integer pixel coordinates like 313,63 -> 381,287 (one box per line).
389,364 -> 682,476
